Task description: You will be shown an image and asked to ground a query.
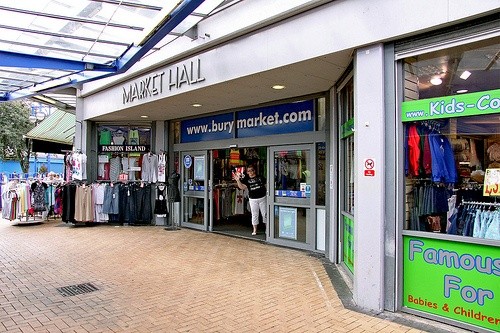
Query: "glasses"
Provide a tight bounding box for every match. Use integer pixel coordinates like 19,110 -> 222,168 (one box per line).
247,169 -> 255,172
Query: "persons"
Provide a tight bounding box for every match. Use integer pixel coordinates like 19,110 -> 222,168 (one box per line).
234,166 -> 268,236
168,169 -> 181,202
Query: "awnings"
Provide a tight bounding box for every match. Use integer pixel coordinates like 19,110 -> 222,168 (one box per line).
23,106 -> 75,154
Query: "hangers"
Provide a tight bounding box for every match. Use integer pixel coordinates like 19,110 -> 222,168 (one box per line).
408,119 -> 441,134
454,201 -> 500,214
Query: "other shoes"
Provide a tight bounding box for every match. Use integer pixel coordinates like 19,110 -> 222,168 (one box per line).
252,232 -> 256,235
